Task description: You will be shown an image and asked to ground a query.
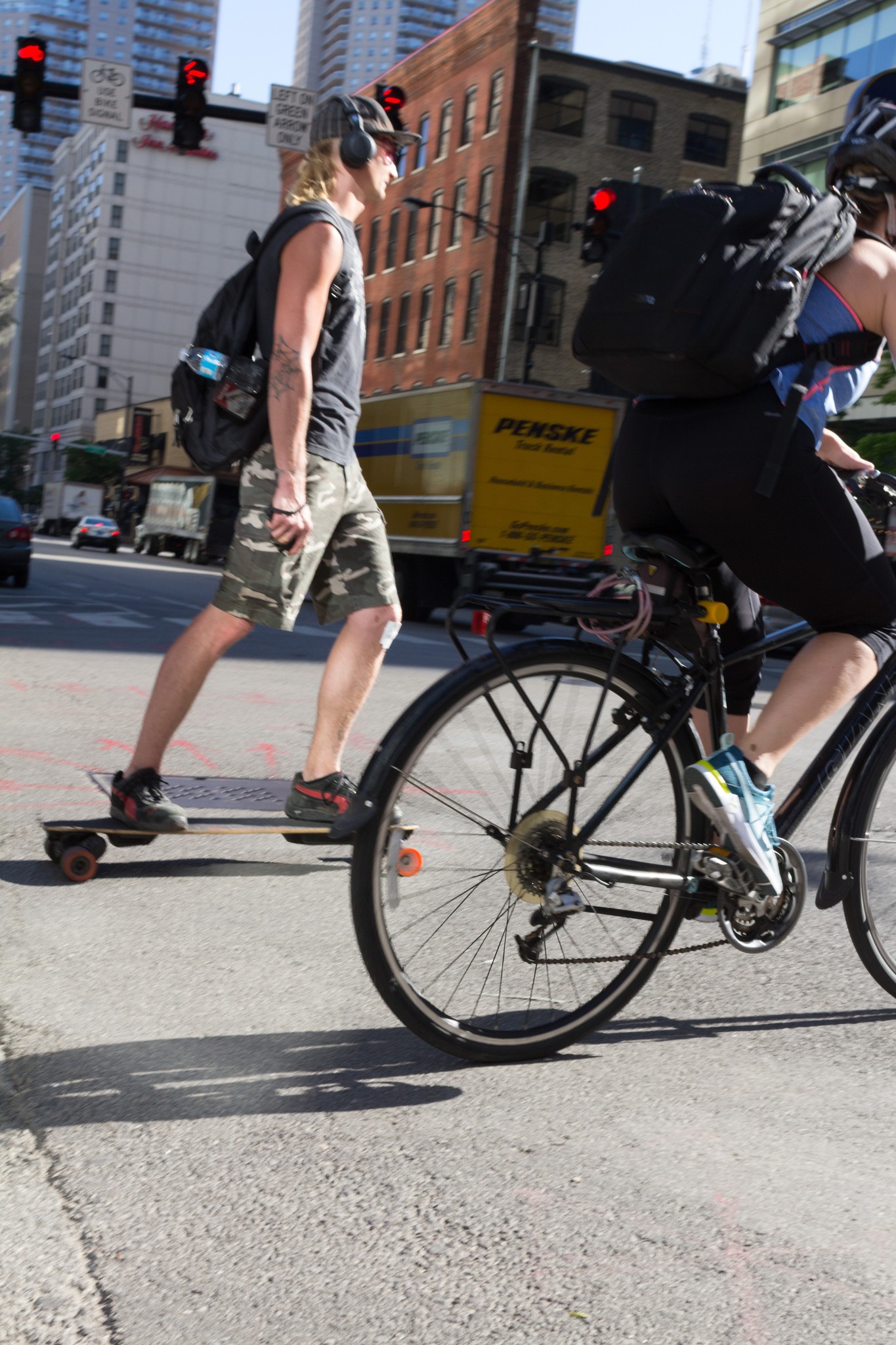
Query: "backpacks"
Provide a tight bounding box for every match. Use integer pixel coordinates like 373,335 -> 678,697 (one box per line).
170,205 -> 353,472
566,159 -> 890,401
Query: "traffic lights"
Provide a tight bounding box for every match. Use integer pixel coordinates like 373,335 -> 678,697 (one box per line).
581,185 -> 617,261
50,433 -> 61,451
177,57 -> 207,147
378,85 -> 406,165
13,37 -> 45,131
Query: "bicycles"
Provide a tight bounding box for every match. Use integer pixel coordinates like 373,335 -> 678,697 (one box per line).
325,460 -> 895,1065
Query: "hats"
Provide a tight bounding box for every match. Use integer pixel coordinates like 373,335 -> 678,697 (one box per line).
307,93 -> 423,149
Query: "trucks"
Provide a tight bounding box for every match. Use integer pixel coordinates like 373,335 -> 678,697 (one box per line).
354,378 -> 627,632
36,479 -> 106,537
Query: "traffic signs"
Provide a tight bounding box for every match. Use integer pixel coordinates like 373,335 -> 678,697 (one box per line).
79,57 -> 135,128
265,83 -> 318,153
84,443 -> 108,454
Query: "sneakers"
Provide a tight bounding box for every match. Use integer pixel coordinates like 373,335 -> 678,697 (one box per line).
109,770 -> 189,835
680,726 -> 783,894
284,770 -> 405,830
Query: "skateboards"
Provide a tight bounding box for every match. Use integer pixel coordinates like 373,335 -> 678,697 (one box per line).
36,813 -> 423,883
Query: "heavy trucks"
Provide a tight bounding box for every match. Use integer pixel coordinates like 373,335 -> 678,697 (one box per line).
133,476 -> 240,565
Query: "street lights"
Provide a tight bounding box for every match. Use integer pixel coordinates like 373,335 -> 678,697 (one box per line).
59,354 -> 135,509
399,196 -> 556,383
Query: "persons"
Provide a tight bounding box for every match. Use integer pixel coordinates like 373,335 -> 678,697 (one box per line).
610,92 -> 896,927
111,94 -> 428,832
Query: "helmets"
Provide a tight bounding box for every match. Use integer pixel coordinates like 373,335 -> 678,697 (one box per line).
824,67 -> 896,202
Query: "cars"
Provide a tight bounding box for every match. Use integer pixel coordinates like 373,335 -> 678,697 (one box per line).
71,515 -> 122,553
0,495 -> 33,588
20,513 -> 40,531
